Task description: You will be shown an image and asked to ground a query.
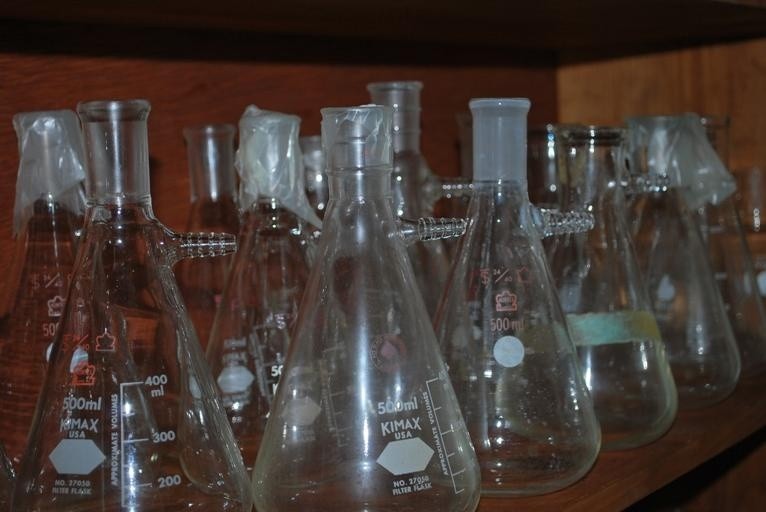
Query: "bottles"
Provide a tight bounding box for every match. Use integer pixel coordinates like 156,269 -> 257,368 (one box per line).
1,78 -> 765,512
